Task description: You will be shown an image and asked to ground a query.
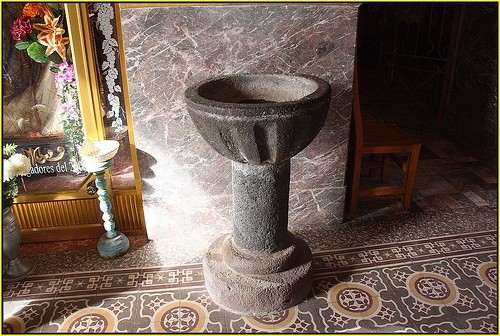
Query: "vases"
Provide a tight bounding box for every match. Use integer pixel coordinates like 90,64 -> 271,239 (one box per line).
2,208 -> 36,283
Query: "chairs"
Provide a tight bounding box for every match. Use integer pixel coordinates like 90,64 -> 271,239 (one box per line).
349,48 -> 422,216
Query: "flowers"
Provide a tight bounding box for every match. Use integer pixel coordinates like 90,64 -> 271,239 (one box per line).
2,143 -> 32,209
